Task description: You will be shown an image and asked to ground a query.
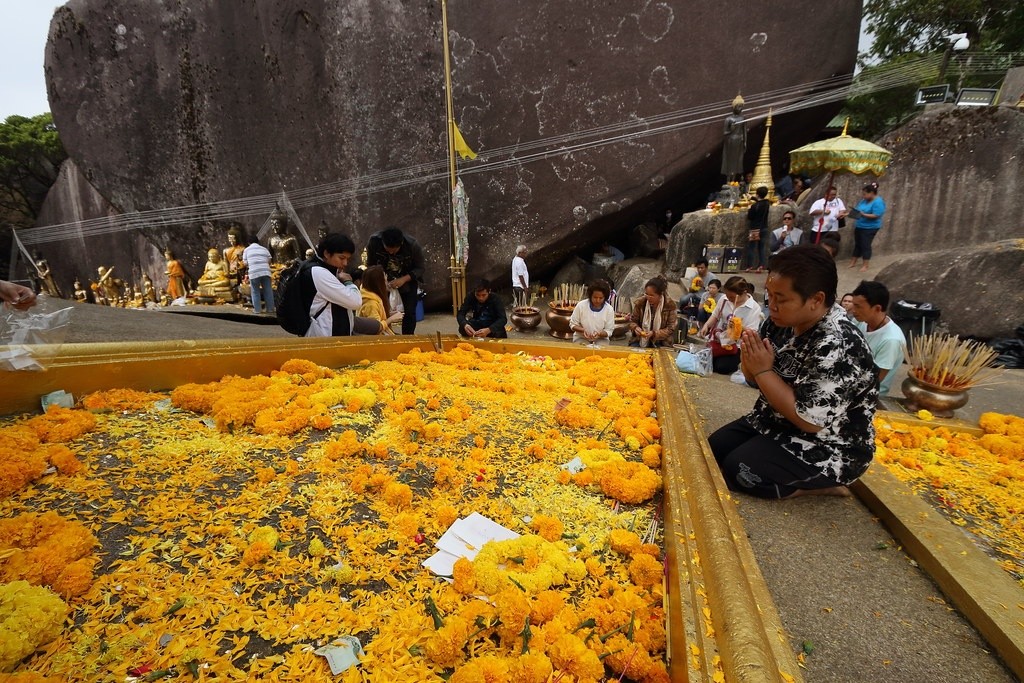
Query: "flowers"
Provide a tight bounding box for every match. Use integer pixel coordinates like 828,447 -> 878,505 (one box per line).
871,410 -> 1024,487
0,342 -> 674,683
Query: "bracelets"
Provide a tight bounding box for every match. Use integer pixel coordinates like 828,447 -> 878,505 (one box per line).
755,370 -> 773,377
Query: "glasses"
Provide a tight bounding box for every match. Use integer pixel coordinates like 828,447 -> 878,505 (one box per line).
783,217 -> 792,220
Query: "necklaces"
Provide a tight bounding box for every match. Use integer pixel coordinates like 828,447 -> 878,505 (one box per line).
799,311 -> 827,335
874,316 -> 886,331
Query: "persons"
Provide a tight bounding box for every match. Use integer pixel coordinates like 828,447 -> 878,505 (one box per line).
810,186 -> 847,245
709,238 -> 879,498
720,94 -> 747,183
841,182 -> 885,272
512,245 -> 529,305
840,281 -> 907,396
32,222 -> 247,309
243,236 -> 274,313
302,227 -> 422,337
568,279 -> 614,346
267,208 -> 300,264
629,257 -> 763,376
746,187 -> 771,272
782,176 -> 812,205
456,278 -> 508,339
743,171 -> 753,191
768,211 -> 804,257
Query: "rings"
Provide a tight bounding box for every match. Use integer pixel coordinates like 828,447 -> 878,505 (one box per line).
396,286 -> 398,288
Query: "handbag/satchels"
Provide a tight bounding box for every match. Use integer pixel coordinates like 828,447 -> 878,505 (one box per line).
672,343 -> 713,376
747,229 -> 760,241
837,217 -> 846,228
389,287 -> 404,313
706,338 -> 738,357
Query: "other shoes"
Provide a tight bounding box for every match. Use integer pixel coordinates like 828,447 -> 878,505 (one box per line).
745,266 -> 752,273
253,309 -> 260,313
268,308 -> 276,312
755,266 -> 764,273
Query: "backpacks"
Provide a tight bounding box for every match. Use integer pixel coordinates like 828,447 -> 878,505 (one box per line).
274,261 -> 330,337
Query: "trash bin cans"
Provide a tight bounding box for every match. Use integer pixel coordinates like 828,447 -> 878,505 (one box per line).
887,298 -> 942,364
703,244 -> 725,273
722,245 -> 745,273
416,287 -> 426,321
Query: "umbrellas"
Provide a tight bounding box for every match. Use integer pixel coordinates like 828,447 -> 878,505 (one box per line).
790,117 -> 895,205
